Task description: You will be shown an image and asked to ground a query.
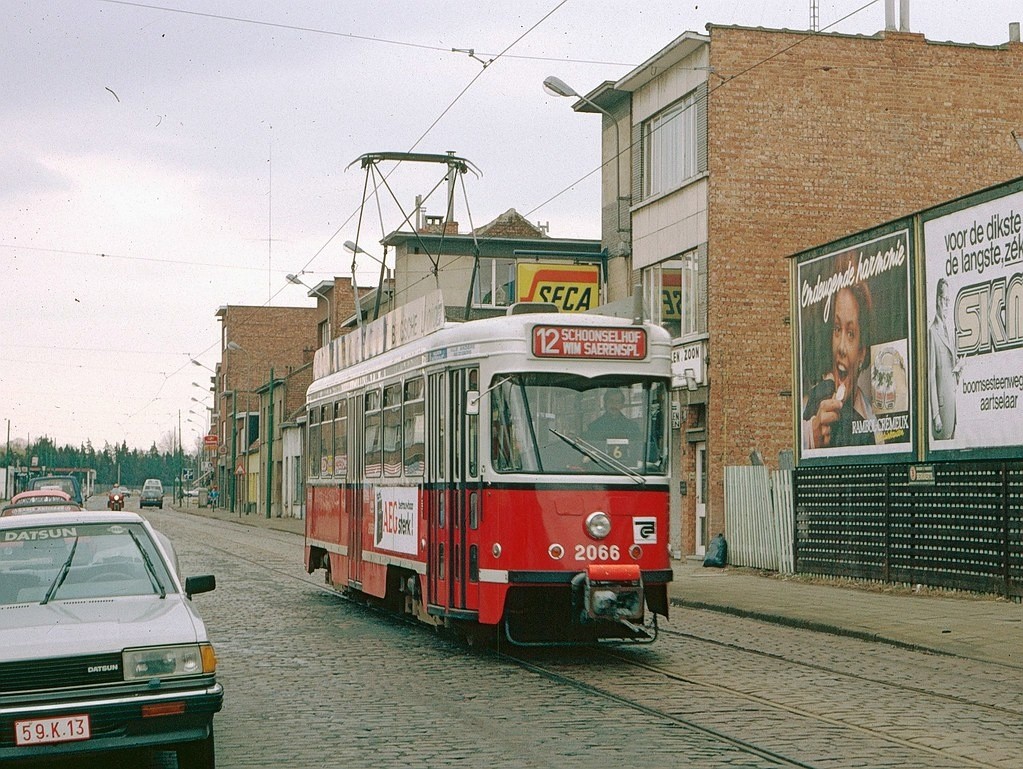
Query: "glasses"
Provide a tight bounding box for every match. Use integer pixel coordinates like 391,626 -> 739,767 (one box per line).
940,296 -> 950,302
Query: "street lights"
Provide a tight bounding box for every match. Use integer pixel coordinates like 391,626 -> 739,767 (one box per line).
188,359 -> 218,513
228,342 -> 251,518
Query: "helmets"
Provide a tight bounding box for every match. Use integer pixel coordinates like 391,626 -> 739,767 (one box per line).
114,484 -> 119,488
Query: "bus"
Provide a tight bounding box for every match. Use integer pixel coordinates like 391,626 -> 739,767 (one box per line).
302,303 -> 699,652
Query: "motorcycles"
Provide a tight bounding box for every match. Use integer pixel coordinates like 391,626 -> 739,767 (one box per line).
106,490 -> 126,512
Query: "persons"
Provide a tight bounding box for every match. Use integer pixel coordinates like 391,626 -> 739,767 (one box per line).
928,279 -> 962,439
802,270 -> 885,450
586,390 -> 642,441
109,483 -> 122,495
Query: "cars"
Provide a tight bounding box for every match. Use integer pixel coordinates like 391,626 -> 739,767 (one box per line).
0,490 -> 224,769
184,487 -> 199,497
81,484 -> 93,501
137,490 -> 165,508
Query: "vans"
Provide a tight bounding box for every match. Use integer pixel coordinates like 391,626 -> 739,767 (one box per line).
143,479 -> 164,495
27,476 -> 83,509
